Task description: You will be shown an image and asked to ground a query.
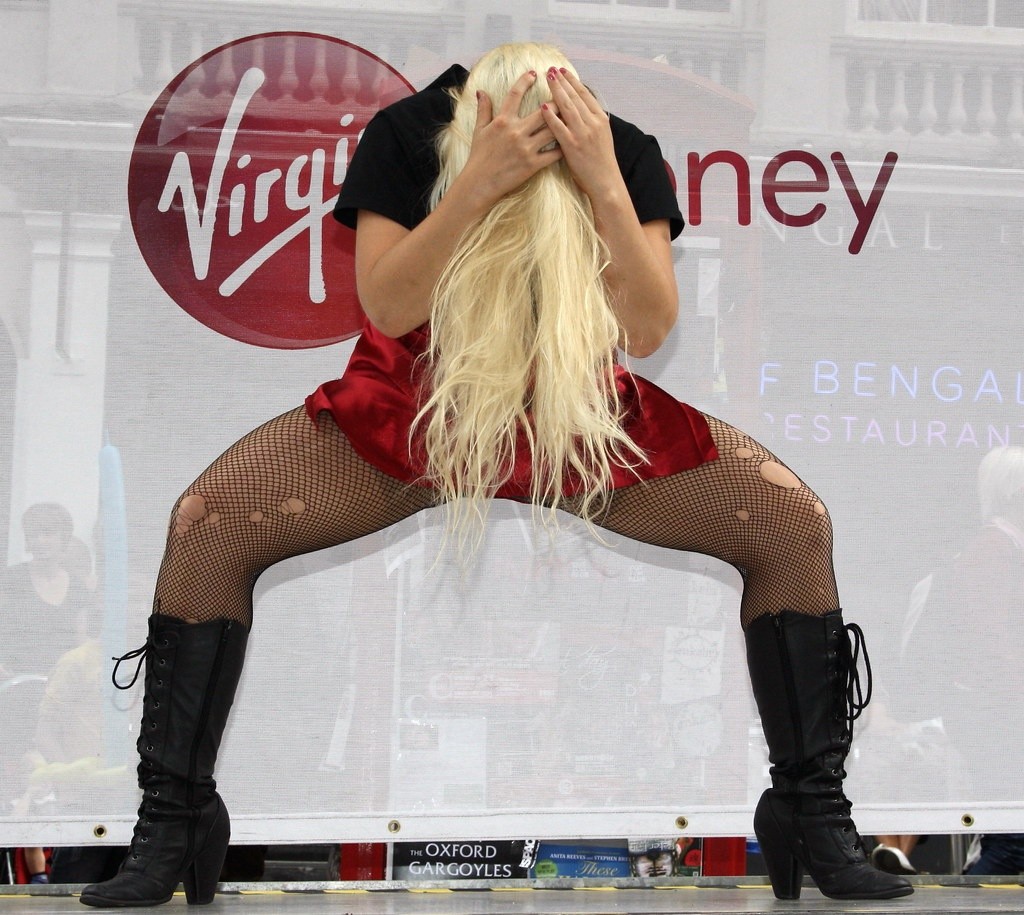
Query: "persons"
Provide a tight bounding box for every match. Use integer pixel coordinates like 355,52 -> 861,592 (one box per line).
0,501 -> 91,884
79,42 -> 915,908
870,833 -> 1024,875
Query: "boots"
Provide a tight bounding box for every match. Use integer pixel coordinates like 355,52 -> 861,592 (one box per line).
745,608 -> 915,901
78,612 -> 251,907
